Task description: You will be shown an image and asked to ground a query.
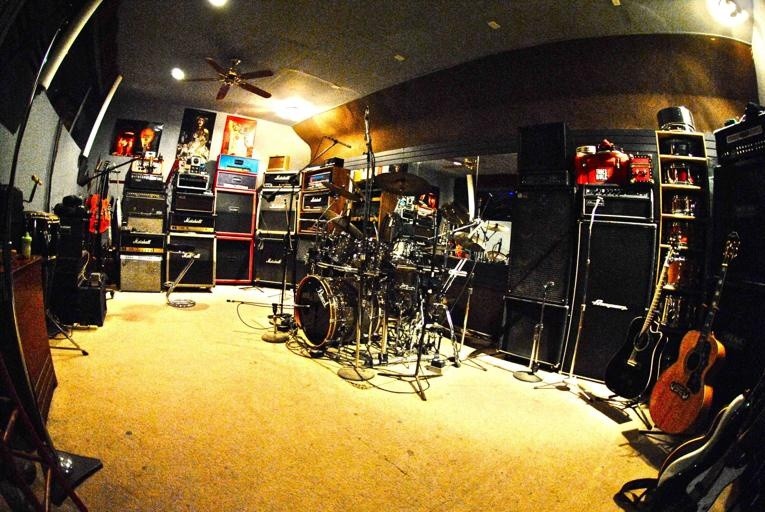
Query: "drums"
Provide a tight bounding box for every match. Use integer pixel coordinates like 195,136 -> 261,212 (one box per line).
296,276 -> 362,347
353,238 -> 388,272
328,232 -> 355,265
390,238 -> 424,272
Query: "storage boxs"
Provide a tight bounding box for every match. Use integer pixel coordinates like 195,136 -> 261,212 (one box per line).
575,151 -> 654,186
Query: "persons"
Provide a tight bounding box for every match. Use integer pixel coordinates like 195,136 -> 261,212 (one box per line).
228,120 -> 247,157
191,116 -> 210,143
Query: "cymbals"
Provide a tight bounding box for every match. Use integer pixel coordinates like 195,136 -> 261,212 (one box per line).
441,203 -> 469,224
322,181 -> 357,200
376,172 -> 429,196
320,206 -> 360,238
455,234 -> 485,251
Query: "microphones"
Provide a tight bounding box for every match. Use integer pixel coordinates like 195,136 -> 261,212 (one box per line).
327,135 -> 352,148
364,111 -> 370,141
32,174 -> 43,185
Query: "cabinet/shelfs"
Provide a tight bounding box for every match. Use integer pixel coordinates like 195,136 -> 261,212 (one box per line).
655,129 -> 710,326
297,167 -> 351,237
345,187 -> 440,241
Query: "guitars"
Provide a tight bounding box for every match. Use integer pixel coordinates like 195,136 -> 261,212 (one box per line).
650,233 -> 741,435
605,232 -> 687,402
86,157 -> 112,233
657,377 -> 765,510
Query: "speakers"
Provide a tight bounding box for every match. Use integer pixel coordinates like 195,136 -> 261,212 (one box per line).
560,219 -> 657,384
711,156 -> 765,284
705,277 -> 765,432
496,295 -> 569,368
120,170 -> 316,292
506,186 -> 577,305
517,121 -> 570,172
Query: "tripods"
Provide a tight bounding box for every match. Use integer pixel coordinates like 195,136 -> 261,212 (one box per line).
534,196 -> 601,404
377,208 -> 487,400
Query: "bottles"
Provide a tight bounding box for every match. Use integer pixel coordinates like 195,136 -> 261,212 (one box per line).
20,231 -> 32,260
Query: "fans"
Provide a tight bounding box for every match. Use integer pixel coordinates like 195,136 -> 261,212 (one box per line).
181,56 -> 273,99
